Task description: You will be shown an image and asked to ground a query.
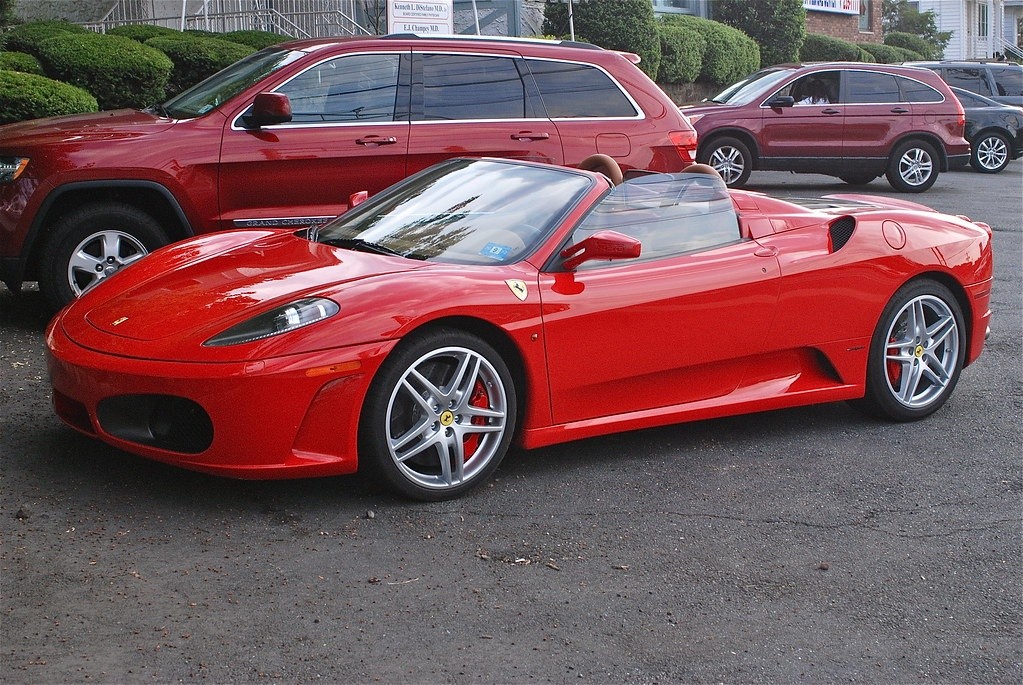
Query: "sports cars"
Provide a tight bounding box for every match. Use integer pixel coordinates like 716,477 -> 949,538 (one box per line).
40,155 -> 993,502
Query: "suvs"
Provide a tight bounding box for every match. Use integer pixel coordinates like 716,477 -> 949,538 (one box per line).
1,34 -> 698,319
887,59 -> 1023,108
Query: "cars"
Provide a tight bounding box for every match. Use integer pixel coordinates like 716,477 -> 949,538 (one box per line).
676,62 -> 970,191
939,85 -> 1023,175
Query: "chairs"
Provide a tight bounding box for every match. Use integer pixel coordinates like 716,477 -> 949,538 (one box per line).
325,68 -> 363,117
644,163 -> 729,256
573,153 -> 626,233
825,82 -> 839,103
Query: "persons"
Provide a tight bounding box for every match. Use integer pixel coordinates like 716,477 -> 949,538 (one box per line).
793,80 -> 830,105
